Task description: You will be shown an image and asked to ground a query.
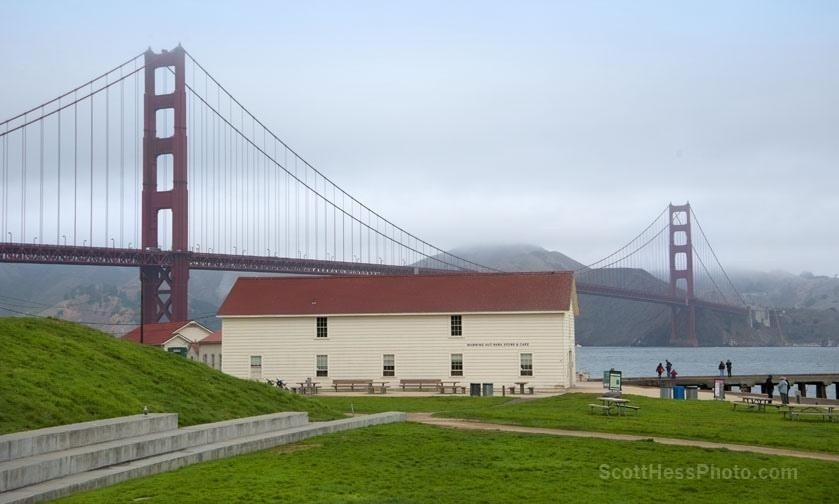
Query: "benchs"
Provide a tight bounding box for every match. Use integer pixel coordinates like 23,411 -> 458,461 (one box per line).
780,409 -> 839,423
292,386 -> 321,395
332,379 -> 439,394
590,404 -> 641,417
733,400 -> 782,412
437,385 -> 465,394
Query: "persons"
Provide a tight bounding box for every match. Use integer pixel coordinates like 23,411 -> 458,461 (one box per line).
765,375 -> 791,405
718,359 -> 734,377
655,360 -> 677,379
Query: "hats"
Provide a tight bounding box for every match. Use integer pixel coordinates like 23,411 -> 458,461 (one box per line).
778,376 -> 786,380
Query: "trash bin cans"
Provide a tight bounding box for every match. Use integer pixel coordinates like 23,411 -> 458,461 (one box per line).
483,383 -> 493,396
470,383 -> 480,396
660,386 -> 698,400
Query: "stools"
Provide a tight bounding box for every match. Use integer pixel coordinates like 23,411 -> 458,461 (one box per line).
507,386 -> 534,394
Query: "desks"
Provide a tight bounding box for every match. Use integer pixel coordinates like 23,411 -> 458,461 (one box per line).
596,396 -> 630,416
739,396 -> 775,412
514,382 -> 527,394
295,382 -> 319,394
787,404 -> 834,422
440,381 -> 461,394
